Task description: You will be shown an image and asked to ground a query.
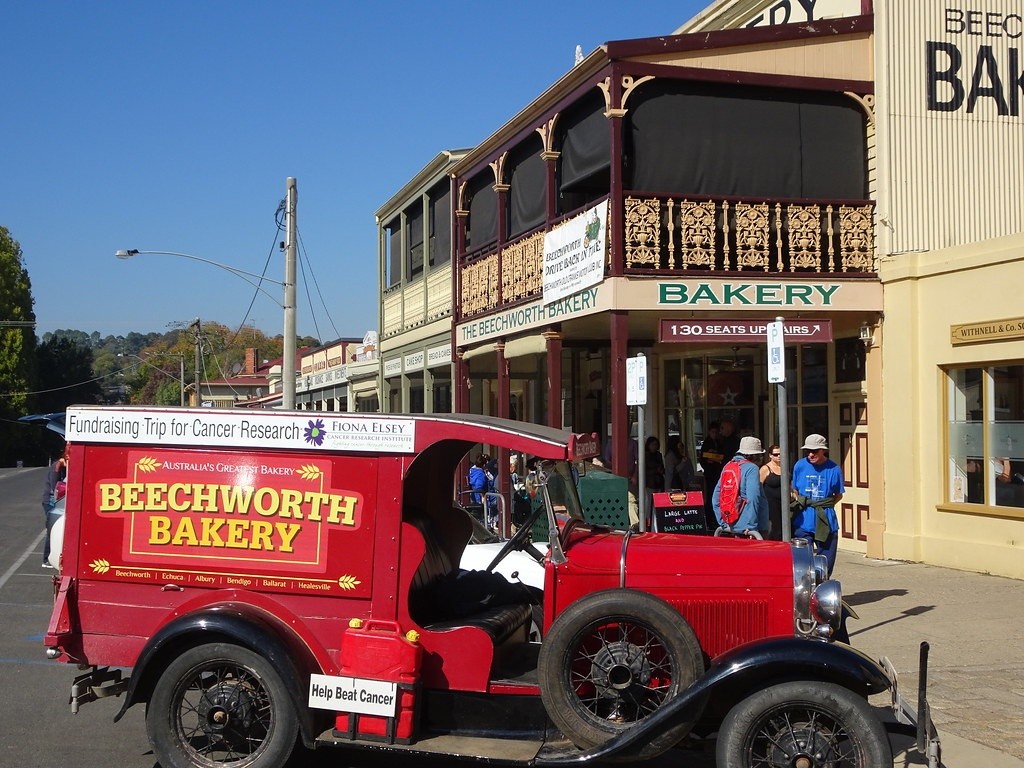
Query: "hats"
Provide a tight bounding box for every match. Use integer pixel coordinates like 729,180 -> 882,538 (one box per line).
800,434 -> 829,449
525,458 -> 537,470
736,436 -> 766,454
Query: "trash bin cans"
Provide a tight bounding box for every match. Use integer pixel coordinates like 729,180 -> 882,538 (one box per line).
531,470 -> 629,542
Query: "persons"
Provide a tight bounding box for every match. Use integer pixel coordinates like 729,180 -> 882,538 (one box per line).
525,457 -> 539,498
712,436 -> 766,536
645,437 -> 666,527
967,456 -> 981,501
469,453 -> 498,534
708,419 -> 740,529
759,445 -> 791,540
605,438 -> 638,490
699,425 -> 720,530
510,455 -> 520,514
792,434 -> 845,578
664,435 -> 694,489
42,460 -> 65,568
996,457 -> 1024,486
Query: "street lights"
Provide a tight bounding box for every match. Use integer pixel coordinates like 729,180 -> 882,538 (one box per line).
116,246 -> 300,408
117,351 -> 201,406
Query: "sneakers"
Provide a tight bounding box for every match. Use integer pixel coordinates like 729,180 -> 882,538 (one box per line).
42,562 -> 53,568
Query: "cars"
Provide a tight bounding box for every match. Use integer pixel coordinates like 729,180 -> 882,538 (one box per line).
18,412 -> 64,572
44,406 -> 942,768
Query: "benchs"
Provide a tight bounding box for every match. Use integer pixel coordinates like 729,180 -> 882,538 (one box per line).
404,507 -> 533,668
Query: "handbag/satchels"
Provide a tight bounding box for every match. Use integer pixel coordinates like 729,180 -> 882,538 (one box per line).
671,465 -> 684,489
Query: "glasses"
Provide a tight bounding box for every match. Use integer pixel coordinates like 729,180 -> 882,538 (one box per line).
771,454 -> 780,456
720,426 -> 726,430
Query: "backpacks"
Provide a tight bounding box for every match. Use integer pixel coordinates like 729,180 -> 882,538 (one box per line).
487,475 -> 498,507
719,459 -> 753,525
